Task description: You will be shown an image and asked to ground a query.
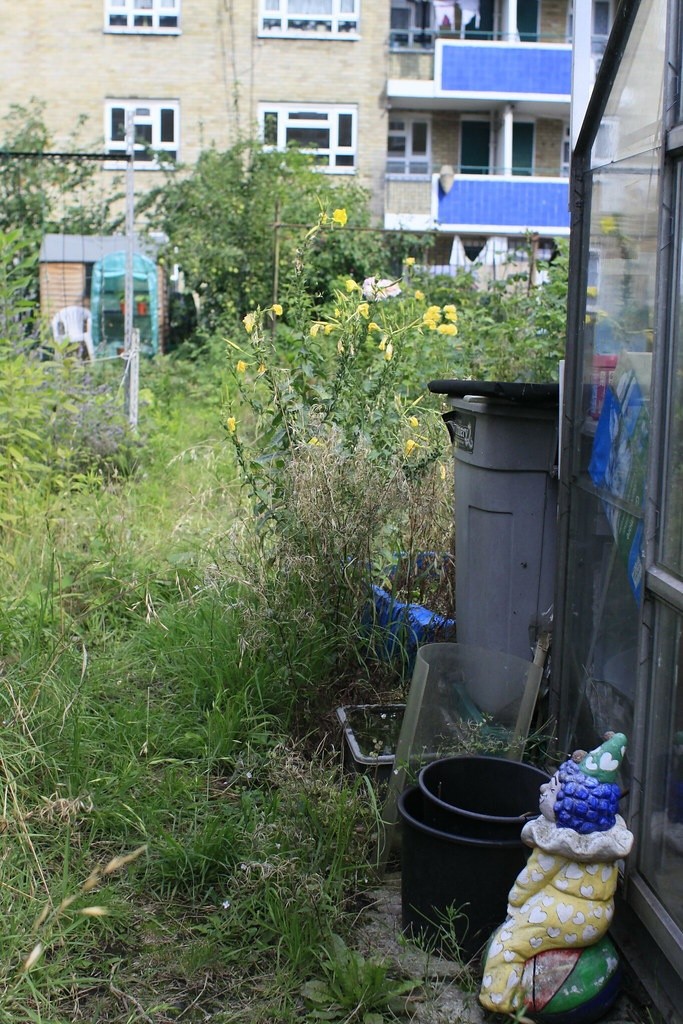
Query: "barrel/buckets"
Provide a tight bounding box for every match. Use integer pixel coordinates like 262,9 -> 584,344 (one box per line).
396,787 -> 534,962
419,754 -> 554,841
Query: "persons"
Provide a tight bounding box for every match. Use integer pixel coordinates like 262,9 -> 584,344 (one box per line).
479,733 -> 634,1013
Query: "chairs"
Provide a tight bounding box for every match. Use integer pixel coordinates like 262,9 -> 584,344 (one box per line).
51,305 -> 96,367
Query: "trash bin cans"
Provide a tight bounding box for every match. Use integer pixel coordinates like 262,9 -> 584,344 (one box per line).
423,371 -> 564,718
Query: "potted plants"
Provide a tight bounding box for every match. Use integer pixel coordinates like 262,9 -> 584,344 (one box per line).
115,290 -> 125,315
136,295 -> 150,315
